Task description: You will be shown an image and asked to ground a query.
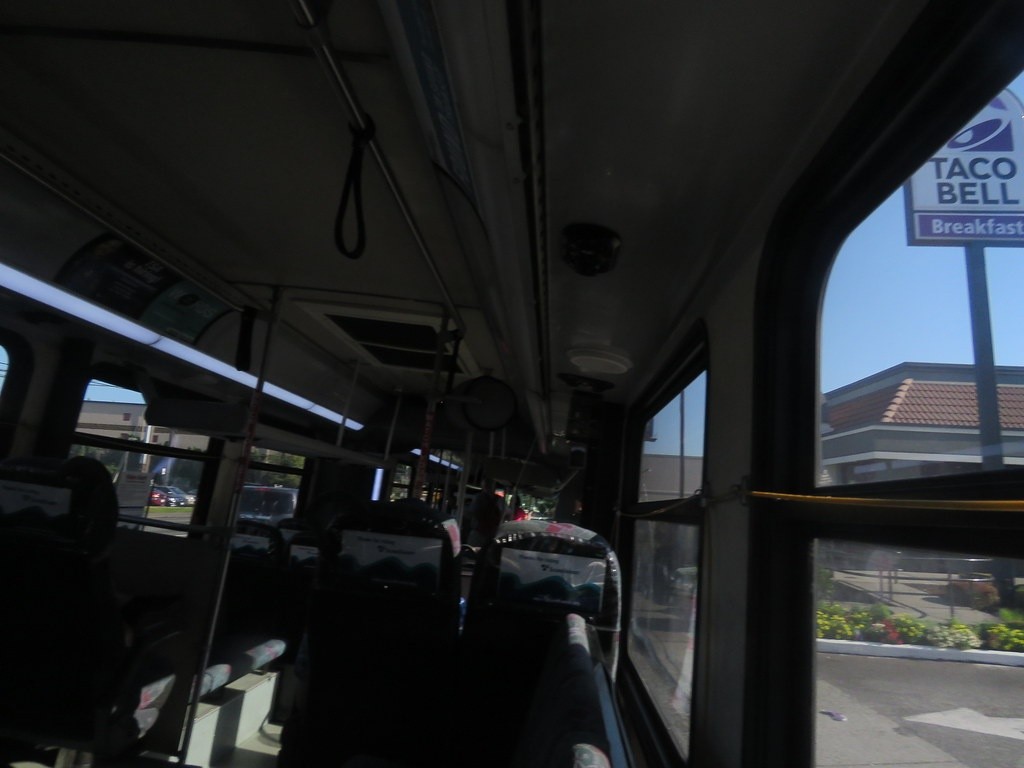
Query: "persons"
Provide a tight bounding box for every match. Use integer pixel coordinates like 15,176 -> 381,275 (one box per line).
45,455 -> 527,739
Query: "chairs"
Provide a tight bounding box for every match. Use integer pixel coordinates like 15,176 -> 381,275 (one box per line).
0,454 -> 639,768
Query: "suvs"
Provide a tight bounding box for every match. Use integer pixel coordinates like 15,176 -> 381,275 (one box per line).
240,481 -> 300,528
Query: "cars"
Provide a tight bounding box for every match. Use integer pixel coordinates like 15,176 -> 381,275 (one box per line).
151,484 -> 198,508
